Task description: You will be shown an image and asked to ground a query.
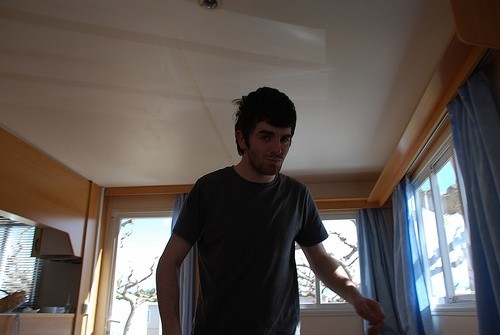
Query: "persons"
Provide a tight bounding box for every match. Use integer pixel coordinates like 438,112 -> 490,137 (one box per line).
156,87 -> 386,334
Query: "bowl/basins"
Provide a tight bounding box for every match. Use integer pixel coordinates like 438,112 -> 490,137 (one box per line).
56,307 -> 66,313
42,307 -> 57,313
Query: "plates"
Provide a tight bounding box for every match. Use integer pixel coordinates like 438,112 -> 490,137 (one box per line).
22,309 -> 40,313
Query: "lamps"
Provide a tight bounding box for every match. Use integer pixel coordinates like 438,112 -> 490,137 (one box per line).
196,0 -> 225,13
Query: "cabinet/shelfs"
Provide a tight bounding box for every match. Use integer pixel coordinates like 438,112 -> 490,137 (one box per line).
30,226 -> 83,264
